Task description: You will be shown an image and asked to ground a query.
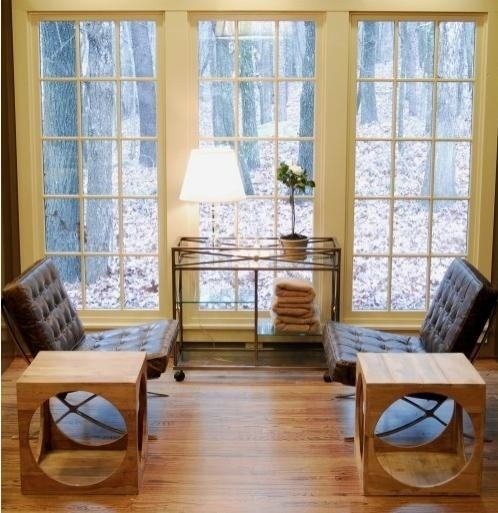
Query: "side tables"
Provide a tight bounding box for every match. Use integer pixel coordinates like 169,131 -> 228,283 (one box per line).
15,349 -> 147,496
353,351 -> 486,495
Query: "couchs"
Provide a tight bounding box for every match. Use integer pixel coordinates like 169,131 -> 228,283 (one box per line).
322,257 -> 497,442
1,254 -> 180,440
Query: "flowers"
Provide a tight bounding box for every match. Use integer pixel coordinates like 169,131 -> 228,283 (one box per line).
276,152 -> 315,235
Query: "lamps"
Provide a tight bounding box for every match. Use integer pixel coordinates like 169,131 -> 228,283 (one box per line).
177,147 -> 247,252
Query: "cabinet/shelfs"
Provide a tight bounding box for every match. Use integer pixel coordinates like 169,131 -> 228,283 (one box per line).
170,235 -> 341,383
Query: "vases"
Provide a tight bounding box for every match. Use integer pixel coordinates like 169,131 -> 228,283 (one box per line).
277,234 -> 308,260
